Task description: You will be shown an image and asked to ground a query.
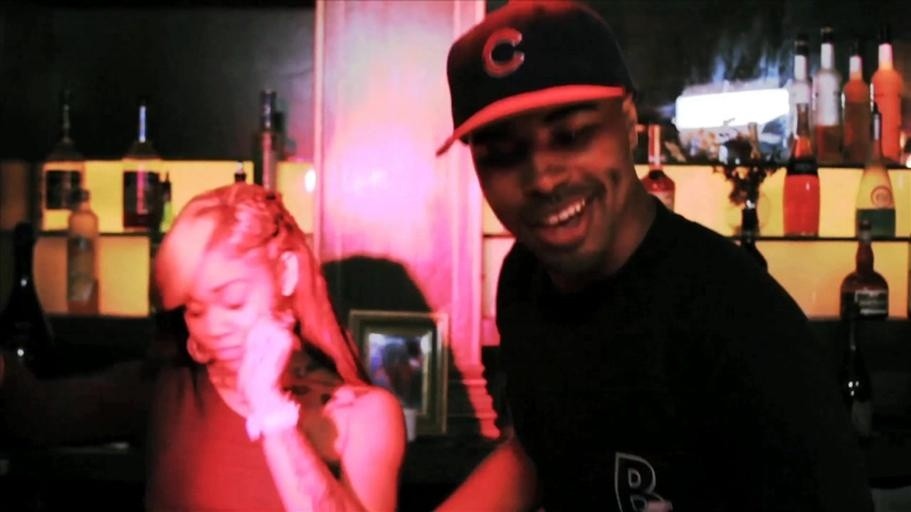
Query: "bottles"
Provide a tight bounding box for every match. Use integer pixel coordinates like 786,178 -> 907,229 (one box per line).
838,219 -> 888,321
837,322 -> 880,442
857,109 -> 897,238
782,103 -> 822,239
790,27 -> 905,161
1,224 -> 53,380
64,188 -> 102,316
31,84 -> 175,229
638,124 -> 674,210
231,161 -> 248,180
250,85 -> 286,189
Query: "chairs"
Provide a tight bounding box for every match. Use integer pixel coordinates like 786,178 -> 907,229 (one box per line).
1,158 -> 315,319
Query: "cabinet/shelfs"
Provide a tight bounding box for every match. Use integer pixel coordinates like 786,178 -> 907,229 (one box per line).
635,159 -> 911,324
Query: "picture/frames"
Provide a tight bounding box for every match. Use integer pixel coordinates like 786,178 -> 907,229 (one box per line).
347,308 -> 449,437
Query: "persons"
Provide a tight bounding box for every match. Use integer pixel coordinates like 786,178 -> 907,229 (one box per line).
432,2 -> 873,510
0,178 -> 408,512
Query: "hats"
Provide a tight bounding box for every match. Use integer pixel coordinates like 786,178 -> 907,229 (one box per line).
434,0 -> 631,157
